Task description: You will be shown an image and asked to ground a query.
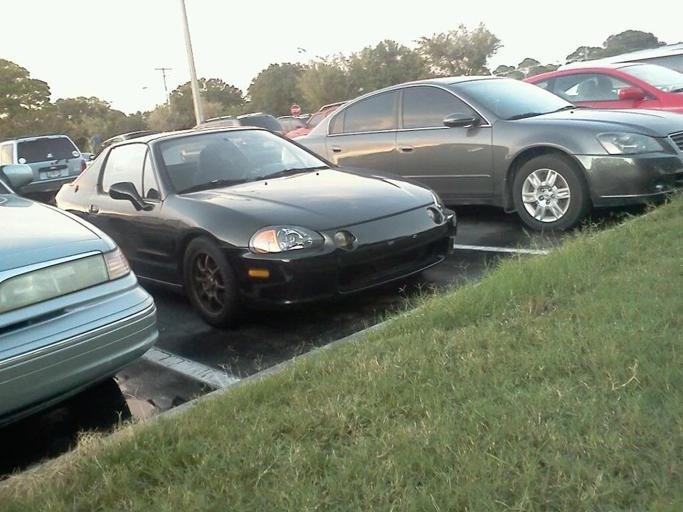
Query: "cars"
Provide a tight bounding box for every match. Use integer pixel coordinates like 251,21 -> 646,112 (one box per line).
0,162 -> 161,427
46,125 -> 458,329
